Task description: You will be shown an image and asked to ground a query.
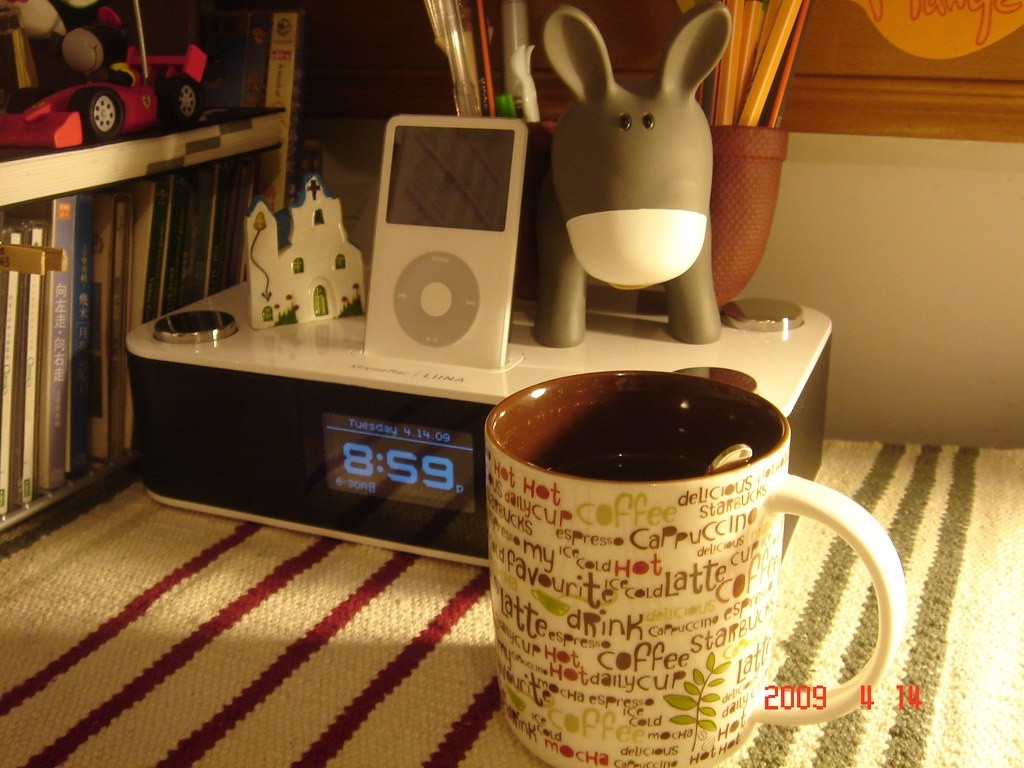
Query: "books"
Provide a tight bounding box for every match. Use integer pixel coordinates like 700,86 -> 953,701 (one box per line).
1,0 -> 312,516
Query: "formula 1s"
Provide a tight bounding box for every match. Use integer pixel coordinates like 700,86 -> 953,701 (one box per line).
1,61 -> 204,149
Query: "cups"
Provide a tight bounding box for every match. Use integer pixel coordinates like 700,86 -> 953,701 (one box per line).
710,127 -> 790,309
486,370 -> 905,768
